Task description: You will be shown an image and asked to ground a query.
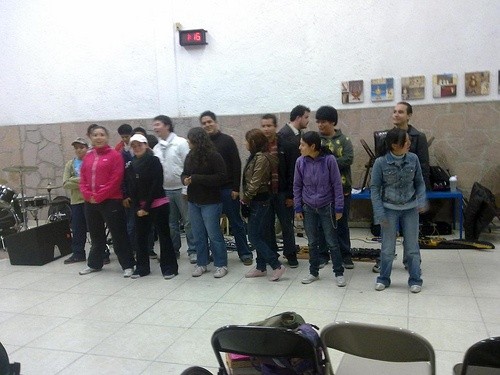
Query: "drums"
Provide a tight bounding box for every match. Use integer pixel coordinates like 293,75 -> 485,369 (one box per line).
19,196 -> 48,211
47,196 -> 73,226
0,185 -> 15,209
0,193 -> 28,230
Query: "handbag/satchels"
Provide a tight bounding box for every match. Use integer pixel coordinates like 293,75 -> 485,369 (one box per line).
48,196 -> 72,225
430,165 -> 451,192
247,311 -> 326,375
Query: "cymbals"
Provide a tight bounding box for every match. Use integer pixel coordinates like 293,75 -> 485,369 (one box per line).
26,185 -> 62,189
2,165 -> 39,173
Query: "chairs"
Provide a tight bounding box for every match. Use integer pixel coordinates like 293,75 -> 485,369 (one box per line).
454,336 -> 500,375
211,323 -> 322,375
320,321 -> 437,375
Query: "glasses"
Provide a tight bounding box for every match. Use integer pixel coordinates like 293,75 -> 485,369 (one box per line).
261,124 -> 273,128
393,109 -> 404,114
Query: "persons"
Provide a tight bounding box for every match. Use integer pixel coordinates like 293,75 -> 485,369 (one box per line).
180,127 -> 229,278
316,106 -> 356,269
240,128 -> 285,281
151,115 -> 198,264
260,113 -> 299,268
293,130 -> 347,287
370,127 -> 428,293
276,104 -> 310,218
371,102 -> 430,273
199,111 -> 253,265
62,124 -> 137,279
113,123 -> 158,259
121,134 -> 179,280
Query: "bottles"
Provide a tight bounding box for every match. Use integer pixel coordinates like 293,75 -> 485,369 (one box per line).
449,178 -> 457,192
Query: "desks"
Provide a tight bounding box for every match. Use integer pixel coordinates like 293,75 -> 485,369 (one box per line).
350,188 -> 463,239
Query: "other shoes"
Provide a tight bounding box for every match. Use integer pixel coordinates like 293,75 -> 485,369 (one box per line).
149,250 -> 158,259
78,266 -> 102,275
288,261 -> 299,268
318,264 -> 325,269
372,263 -> 382,273
131,269 -> 143,278
63,255 -> 86,264
300,274 -> 319,284
104,255 -> 110,264
163,274 -> 175,279
336,275 -> 346,287
269,265 -> 285,281
245,269 -> 268,278
189,253 -> 197,264
243,259 -> 252,265
123,268 -> 134,277
410,284 -> 422,293
375,283 -> 385,291
345,265 -> 354,269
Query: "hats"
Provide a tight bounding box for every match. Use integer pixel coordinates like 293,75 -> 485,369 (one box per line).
71,137 -> 89,145
129,133 -> 148,146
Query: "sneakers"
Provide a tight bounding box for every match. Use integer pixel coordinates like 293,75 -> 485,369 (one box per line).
213,266 -> 228,278
192,264 -> 207,277
